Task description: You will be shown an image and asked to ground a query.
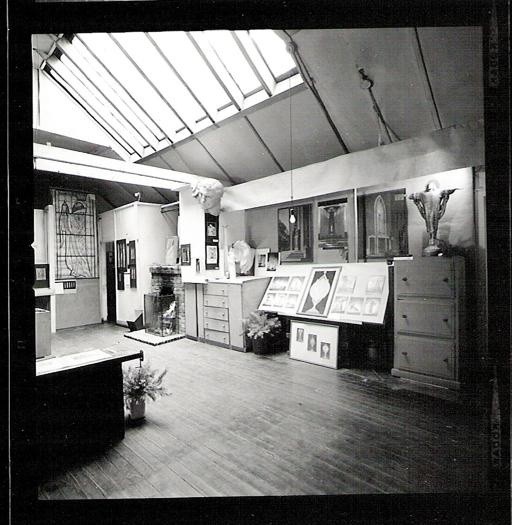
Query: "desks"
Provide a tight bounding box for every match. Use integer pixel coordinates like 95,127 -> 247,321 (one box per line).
36,343 -> 141,473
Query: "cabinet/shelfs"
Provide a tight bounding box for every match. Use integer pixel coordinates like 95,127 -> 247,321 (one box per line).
204,279 -> 273,352
395,257 -> 473,390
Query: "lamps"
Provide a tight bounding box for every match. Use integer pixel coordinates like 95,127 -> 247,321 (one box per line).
288,43 -> 296,223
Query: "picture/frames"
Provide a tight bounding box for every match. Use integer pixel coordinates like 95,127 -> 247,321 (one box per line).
181,244 -> 191,266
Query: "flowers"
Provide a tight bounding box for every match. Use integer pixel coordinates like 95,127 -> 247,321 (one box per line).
243,311 -> 281,339
123,363 -> 171,409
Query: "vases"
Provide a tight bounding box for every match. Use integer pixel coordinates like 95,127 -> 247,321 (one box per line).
250,337 -> 280,354
130,401 -> 145,419
